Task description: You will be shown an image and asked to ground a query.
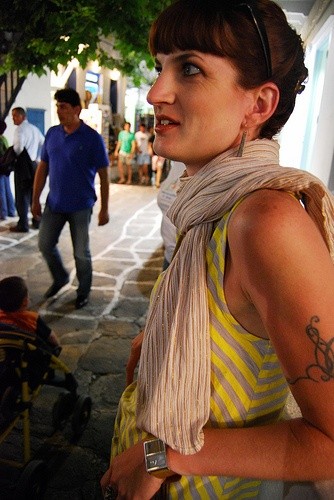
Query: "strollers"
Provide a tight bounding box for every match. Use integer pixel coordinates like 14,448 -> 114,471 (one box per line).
0,326 -> 94,493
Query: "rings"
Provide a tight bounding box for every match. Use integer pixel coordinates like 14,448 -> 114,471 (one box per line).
105,485 -> 117,500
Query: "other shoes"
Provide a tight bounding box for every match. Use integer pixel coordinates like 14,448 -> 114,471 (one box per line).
10,227 -> 28,232
45,276 -> 69,298
33,223 -> 39,228
75,295 -> 88,309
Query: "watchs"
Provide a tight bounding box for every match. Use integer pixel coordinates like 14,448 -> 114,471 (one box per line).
143,434 -> 181,479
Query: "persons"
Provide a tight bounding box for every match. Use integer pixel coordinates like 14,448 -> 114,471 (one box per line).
135,124 -> 167,187
0,118 -> 16,221
32,86 -> 110,308
0,276 -> 62,377
114,122 -> 136,185
100,0 -> 334,500
8,106 -> 45,232
